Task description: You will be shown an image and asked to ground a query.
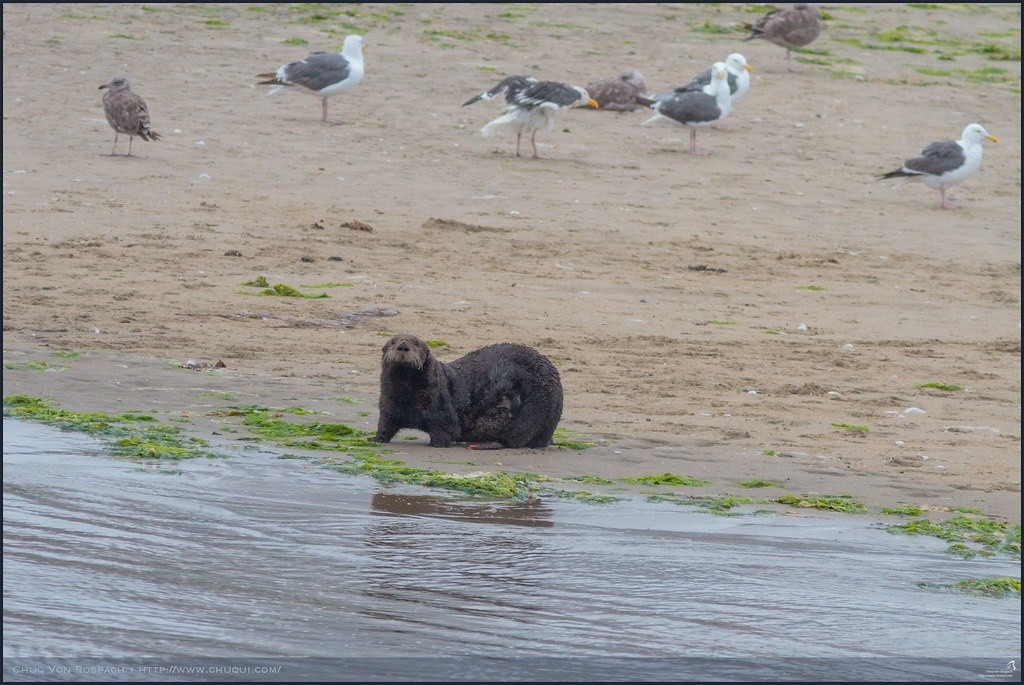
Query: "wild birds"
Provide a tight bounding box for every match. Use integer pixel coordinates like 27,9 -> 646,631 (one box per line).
586,69 -> 650,113
96,73 -> 164,159
461,75 -> 600,159
739,3 -> 823,74
640,52 -> 752,155
874,122 -> 1001,210
255,34 -> 368,125
